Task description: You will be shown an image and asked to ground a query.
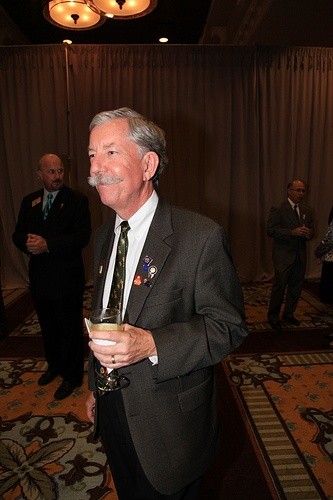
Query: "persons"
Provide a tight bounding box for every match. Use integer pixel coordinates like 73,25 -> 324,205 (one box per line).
265,180 -> 315,330
85,108 -> 247,500
12,154 -> 92,400
314,206 -> 333,347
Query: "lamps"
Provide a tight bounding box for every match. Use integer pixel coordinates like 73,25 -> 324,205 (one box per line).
43,0 -> 158,30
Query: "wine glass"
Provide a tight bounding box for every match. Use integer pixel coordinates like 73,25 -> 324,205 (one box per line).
300,212 -> 308,228
87,307 -> 130,391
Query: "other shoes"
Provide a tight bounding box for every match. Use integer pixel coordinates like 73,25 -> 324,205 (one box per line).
268,320 -> 282,331
282,315 -> 299,326
54,378 -> 81,401
37,370 -> 59,386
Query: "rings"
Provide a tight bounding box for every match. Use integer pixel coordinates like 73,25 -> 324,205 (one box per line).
112,355 -> 115,364
29,249 -> 31,252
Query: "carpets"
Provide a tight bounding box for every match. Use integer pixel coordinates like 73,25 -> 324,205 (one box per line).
0,357 -> 119,500
238,281 -> 333,332
223,349 -> 333,500
0,286 -> 93,337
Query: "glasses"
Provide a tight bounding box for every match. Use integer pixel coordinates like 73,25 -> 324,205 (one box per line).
292,189 -> 306,193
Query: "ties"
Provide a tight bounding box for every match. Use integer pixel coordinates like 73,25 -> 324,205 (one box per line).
294,205 -> 300,225
96,220 -> 133,396
42,194 -> 54,220
